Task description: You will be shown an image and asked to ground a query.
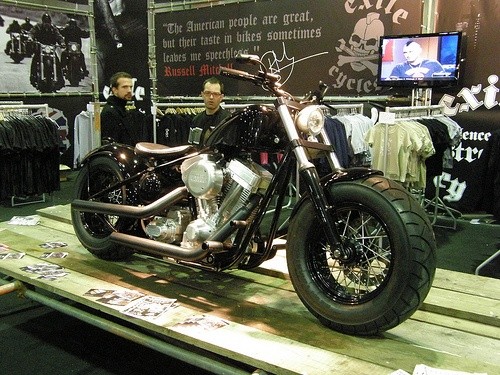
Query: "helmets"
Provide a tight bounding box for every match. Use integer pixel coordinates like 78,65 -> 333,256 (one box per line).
69,19 -> 77,25
42,13 -> 51,22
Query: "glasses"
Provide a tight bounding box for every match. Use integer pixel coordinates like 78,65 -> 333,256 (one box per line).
202,91 -> 222,98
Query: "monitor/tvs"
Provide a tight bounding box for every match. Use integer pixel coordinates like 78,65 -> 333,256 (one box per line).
377,31 -> 462,88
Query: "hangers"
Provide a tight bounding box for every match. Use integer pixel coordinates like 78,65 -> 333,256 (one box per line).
157,108 -> 205,116
0,109 -> 45,119
335,108 -> 359,117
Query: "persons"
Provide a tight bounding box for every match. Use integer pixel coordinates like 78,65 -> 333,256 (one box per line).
187,78 -> 233,145
0,13 -> 90,87
100,72 -> 152,146
388,41 -> 445,76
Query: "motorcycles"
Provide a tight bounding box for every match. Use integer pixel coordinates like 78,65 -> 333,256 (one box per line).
7,30 -> 27,65
28,38 -> 68,93
59,41 -> 88,88
22,29 -> 34,58
70,50 -> 439,337
